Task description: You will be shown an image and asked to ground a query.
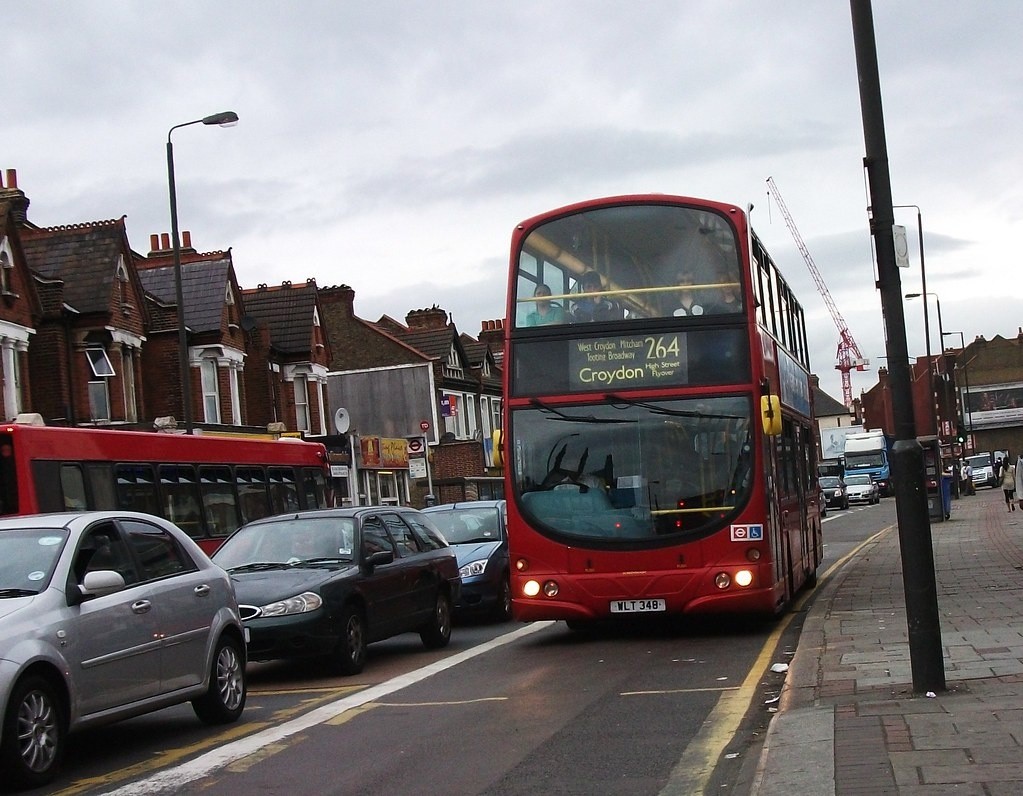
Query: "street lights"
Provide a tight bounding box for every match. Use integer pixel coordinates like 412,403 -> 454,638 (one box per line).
167,110 -> 239,434
867,206 -> 930,356
905,293 -> 960,499
942,332 -> 975,456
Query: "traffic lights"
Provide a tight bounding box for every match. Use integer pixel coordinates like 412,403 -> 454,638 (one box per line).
958,429 -> 966,443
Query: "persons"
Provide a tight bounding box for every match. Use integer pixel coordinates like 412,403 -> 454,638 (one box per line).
717,439 -> 751,508
994,455 -> 1023,511
711,273 -> 744,314
524,284 -> 565,327
569,272 -> 621,325
963,460 -> 976,496
664,270 -> 707,316
554,454 -> 608,494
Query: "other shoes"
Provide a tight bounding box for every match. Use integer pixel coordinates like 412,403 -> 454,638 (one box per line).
1011,503 -> 1015,511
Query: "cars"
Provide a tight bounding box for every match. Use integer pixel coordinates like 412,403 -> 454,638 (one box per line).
959,455 -> 998,488
843,474 -> 880,505
210,506 -> 464,676
0,510 -> 247,787
818,475 -> 849,510
396,499 -> 510,620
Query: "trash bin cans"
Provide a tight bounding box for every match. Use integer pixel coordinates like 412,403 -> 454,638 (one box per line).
942,475 -> 952,519
926,487 -> 942,518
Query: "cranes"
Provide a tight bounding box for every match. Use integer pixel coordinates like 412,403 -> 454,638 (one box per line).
767,176 -> 871,407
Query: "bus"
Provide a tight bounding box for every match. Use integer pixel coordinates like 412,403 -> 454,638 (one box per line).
818,463 -> 844,477
493,194 -> 824,631
0,412 -> 345,559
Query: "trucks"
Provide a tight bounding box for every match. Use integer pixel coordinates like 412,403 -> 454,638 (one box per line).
838,432 -> 895,496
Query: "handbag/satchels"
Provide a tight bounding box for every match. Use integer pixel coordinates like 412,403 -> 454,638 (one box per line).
998,467 -> 1004,486
